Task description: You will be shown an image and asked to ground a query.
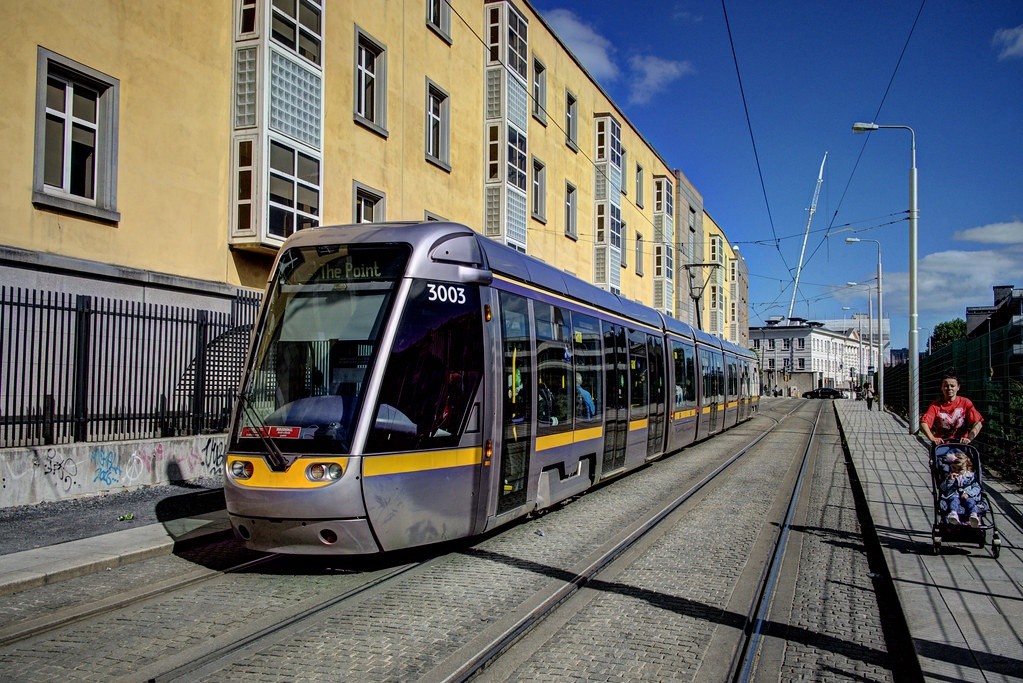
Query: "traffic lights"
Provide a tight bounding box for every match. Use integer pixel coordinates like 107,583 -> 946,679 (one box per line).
784,376 -> 787,382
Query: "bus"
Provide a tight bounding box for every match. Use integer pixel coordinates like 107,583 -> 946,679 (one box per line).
223,220 -> 761,556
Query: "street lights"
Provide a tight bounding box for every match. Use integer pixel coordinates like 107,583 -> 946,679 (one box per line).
846,282 -> 874,389
852,122 -> 920,436
842,307 -> 862,375
845,237 -> 884,412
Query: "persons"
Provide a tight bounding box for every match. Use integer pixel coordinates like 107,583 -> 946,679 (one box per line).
774,385 -> 778,397
764,385 -> 767,393
921,375 -> 984,445
516,373 -> 595,421
939,453 -> 981,528
379,349 -> 438,437
867,385 -> 874,411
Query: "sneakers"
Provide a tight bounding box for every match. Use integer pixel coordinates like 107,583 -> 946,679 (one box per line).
968,516 -> 979,528
949,514 -> 960,526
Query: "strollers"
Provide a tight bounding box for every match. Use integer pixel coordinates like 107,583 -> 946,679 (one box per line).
929,439 -> 1002,559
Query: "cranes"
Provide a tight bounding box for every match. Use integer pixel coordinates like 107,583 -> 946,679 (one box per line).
786,150 -> 828,327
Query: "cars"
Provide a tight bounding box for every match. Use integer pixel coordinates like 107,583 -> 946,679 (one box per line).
801,388 -> 843,400
